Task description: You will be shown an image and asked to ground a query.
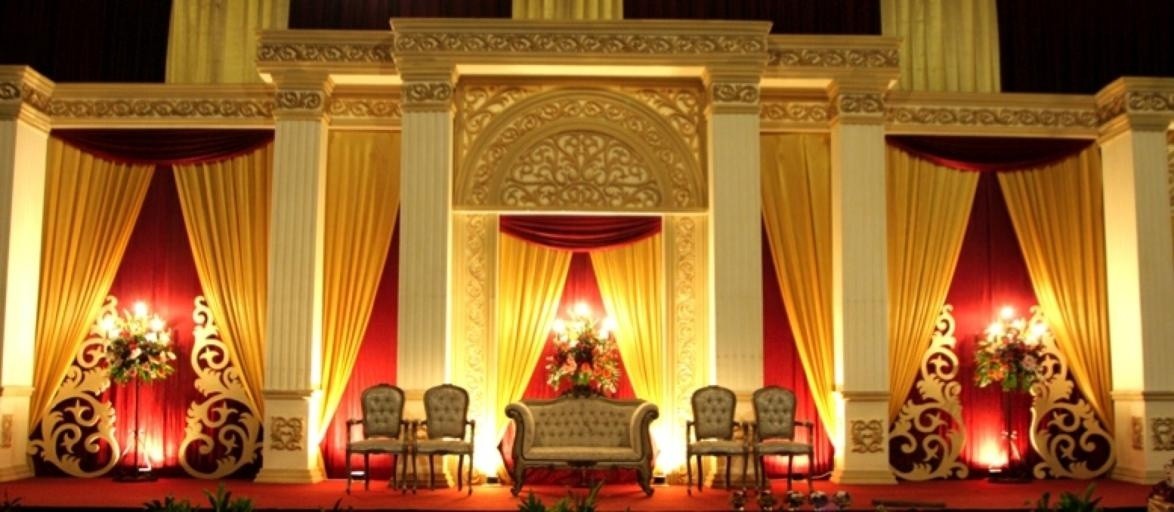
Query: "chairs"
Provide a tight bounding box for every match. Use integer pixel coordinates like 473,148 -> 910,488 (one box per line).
686,385 -> 749,497
750,385 -> 814,492
411,384 -> 475,495
345,384 -> 409,496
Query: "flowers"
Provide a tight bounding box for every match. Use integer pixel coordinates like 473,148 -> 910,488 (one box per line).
974,328 -> 1044,393
105,319 -> 177,388
545,318 -> 621,399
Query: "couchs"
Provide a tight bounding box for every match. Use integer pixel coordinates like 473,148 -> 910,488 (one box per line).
504,386 -> 659,497
